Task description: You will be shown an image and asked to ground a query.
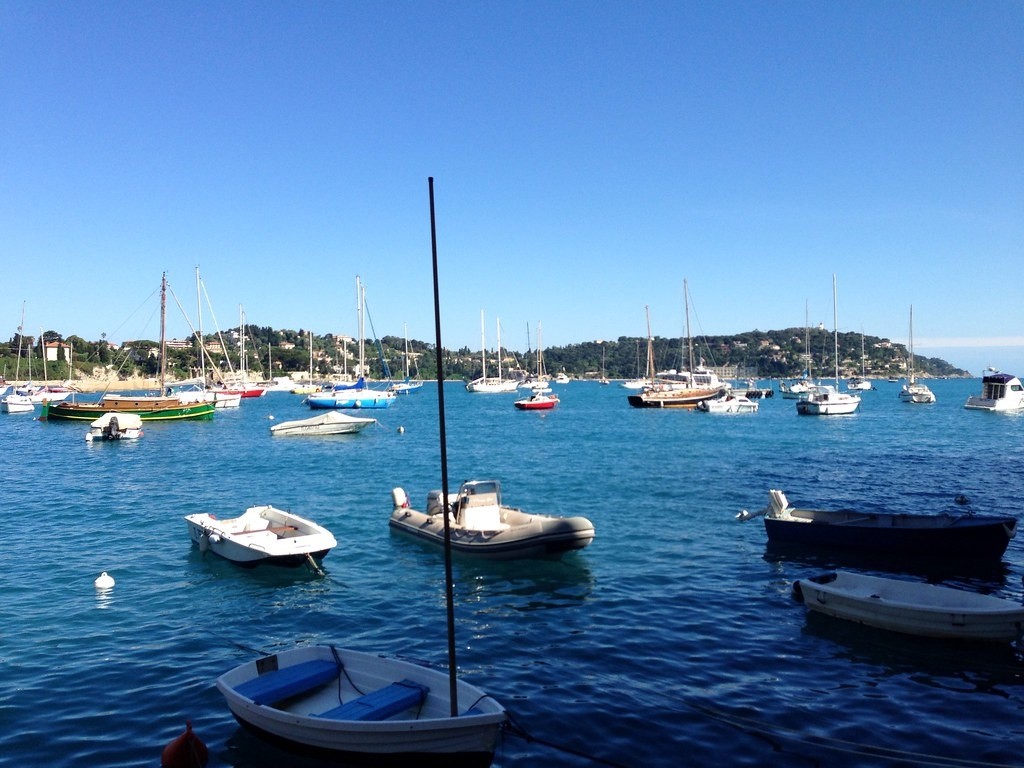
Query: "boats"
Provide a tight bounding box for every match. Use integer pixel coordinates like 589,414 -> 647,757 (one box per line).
697,395 -> 759,414
86,412 -> 142,442
269,411 -> 376,436
555,373 -> 569,384
791,570 -> 1024,643
184,505 -> 338,576
888,376 -> 899,383
257,342 -> 294,392
964,367 -> 1024,411
514,392 -> 560,410
388,479 -> 596,560
734,490 -> 1017,566
718,376 -> 774,399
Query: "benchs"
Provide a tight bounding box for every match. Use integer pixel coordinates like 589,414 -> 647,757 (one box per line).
951,597 -> 975,608
233,658 -> 344,706
852,585 -> 886,596
231,525 -> 298,536
308,679 -> 430,721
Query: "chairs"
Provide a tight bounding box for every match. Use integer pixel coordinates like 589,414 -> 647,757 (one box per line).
457,480 -> 510,531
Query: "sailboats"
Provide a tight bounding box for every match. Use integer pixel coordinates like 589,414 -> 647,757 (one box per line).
783,299 -> 818,398
621,279 -> 732,408
291,332 -> 322,394
847,330 -> 872,389
176,266 -> 241,408
465,309 -> 552,392
307,287 -> 396,409
16,327 -> 77,401
796,274 -> 861,415
599,347 -> 609,385
216,177 -> 508,768
389,324 -> 423,394
48,271 -> 219,421
205,303 -> 264,397
0,300 -> 35,415
899,304 -> 936,402
